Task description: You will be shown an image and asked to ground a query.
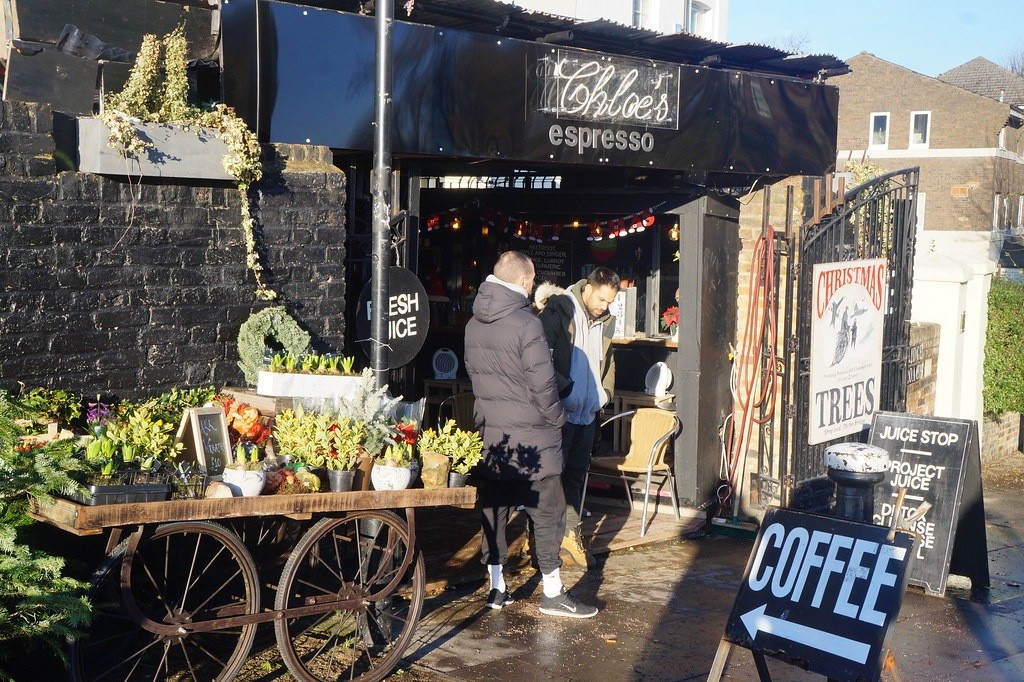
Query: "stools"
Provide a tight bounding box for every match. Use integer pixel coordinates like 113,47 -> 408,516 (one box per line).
619,392 -> 676,456
458,377 -> 477,434
422,376 -> 458,436
599,389 -> 633,453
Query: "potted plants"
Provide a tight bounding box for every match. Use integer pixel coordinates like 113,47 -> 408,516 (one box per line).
0,351 -> 485,506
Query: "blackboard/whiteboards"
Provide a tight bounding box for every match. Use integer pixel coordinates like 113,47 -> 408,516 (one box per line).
869,408 -> 975,599
520,237 -> 573,301
189,406 -> 233,483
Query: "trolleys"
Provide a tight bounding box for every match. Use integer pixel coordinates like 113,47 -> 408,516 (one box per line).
19,485 -> 476,682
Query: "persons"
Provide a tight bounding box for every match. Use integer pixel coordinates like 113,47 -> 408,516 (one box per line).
464,251 -> 598,618
538,266 -> 620,568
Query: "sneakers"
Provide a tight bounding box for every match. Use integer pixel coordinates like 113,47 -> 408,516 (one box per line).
559,527 -> 597,570
519,538 -> 532,560
484,588 -> 515,610
539,585 -> 598,618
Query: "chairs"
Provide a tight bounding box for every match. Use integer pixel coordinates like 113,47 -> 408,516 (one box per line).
437,392 -> 476,437
579,406 -> 681,538
